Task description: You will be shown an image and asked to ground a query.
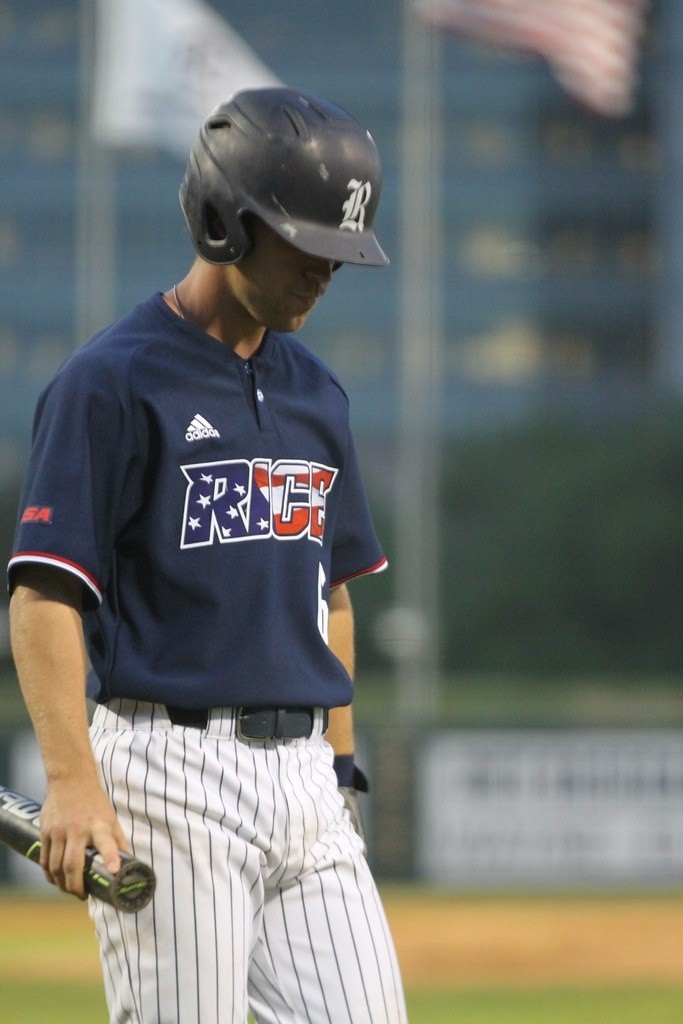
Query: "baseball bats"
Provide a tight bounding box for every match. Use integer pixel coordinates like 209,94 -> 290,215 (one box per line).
0,782 -> 155,914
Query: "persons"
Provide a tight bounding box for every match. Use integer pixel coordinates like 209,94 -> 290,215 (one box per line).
0,85 -> 412,1024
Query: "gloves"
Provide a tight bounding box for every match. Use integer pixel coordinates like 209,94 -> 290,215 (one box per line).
336,753 -> 369,845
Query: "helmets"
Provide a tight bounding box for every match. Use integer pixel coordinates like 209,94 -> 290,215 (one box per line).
177,86 -> 391,268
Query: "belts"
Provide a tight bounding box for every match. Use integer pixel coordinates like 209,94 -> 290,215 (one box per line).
166,702 -> 332,748
